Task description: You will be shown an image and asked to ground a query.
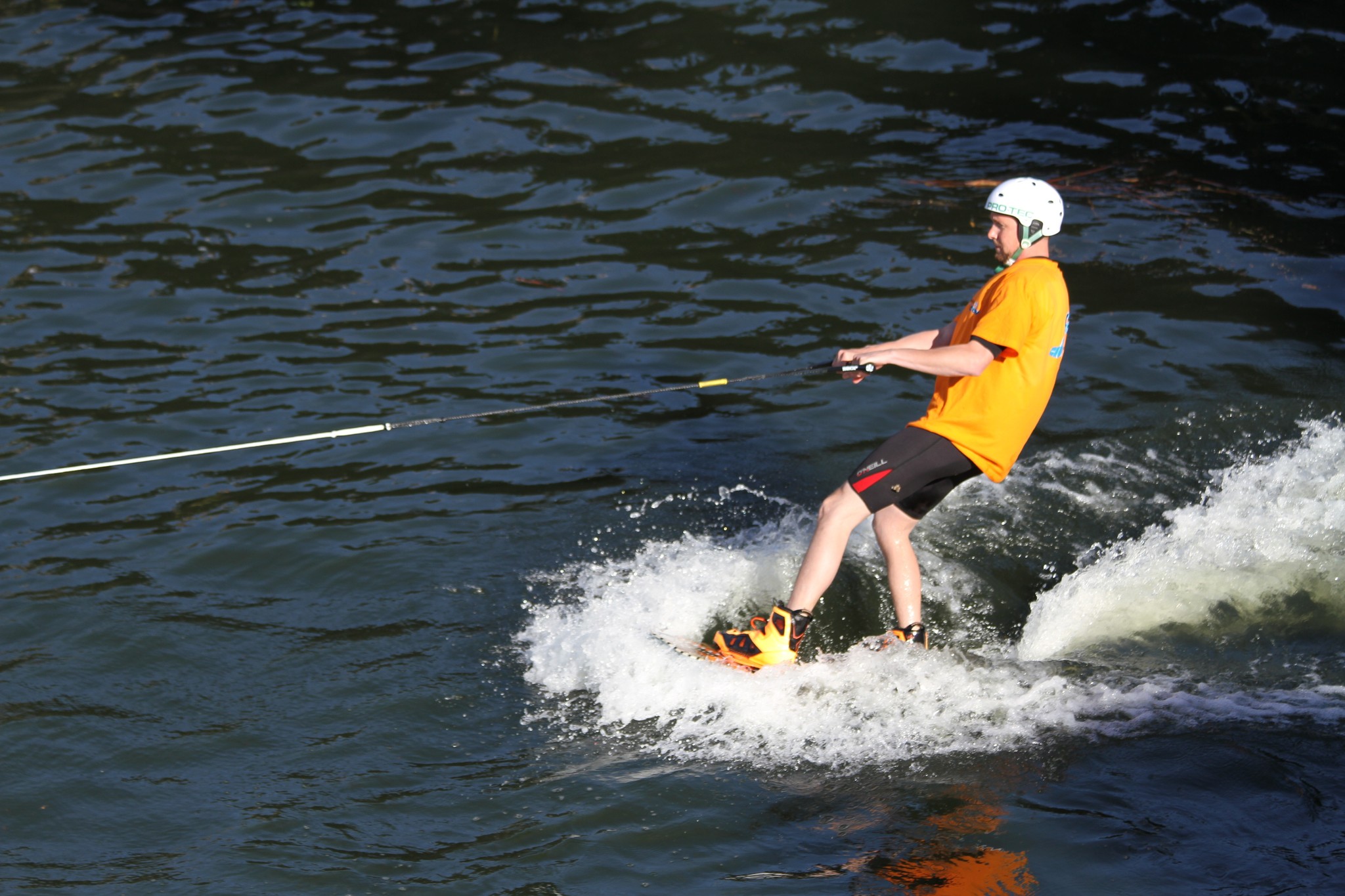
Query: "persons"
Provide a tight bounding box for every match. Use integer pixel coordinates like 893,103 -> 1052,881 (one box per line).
698,176 -> 1071,667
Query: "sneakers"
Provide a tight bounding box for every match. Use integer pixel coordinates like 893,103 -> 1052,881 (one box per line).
702,598 -> 814,670
884,624 -> 930,655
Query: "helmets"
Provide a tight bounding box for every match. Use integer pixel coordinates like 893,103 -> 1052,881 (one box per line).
985,177 -> 1065,238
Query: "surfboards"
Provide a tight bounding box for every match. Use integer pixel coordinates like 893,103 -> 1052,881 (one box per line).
637,618 -> 931,675
